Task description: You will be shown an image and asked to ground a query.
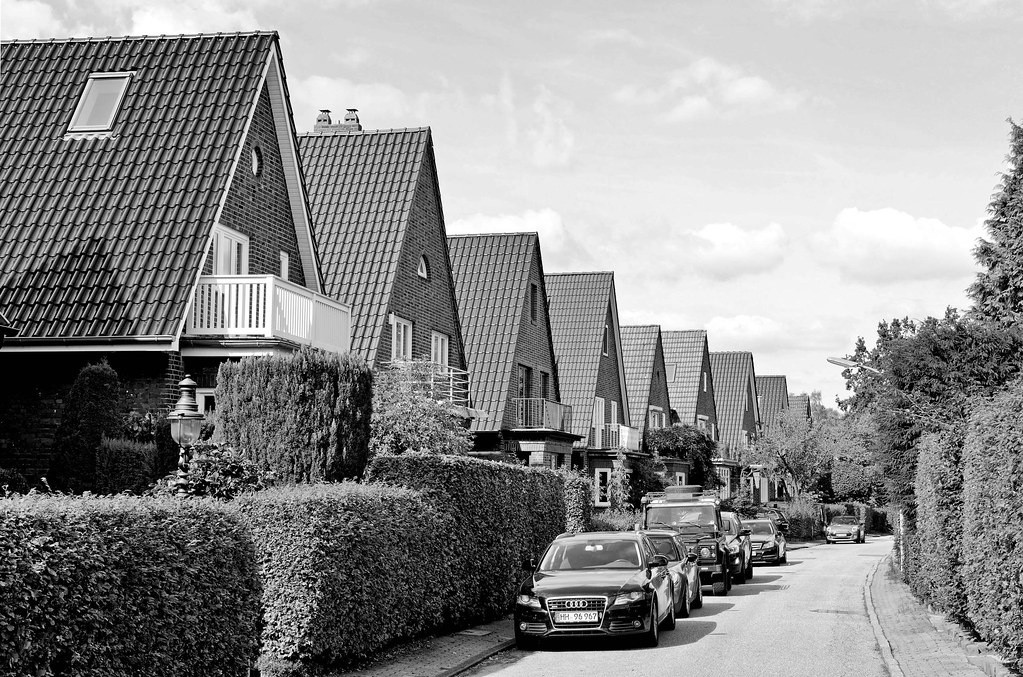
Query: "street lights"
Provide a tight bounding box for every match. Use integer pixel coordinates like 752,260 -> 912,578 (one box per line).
167,372 -> 205,498
825,356 -> 905,575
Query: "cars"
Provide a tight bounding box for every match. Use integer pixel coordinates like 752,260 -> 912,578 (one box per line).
604,528 -> 703,619
740,520 -> 787,567
513,530 -> 676,651
677,511 -> 753,585
825,516 -> 865,544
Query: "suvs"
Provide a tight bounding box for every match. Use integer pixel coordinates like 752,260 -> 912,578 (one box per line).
641,487 -> 732,596
